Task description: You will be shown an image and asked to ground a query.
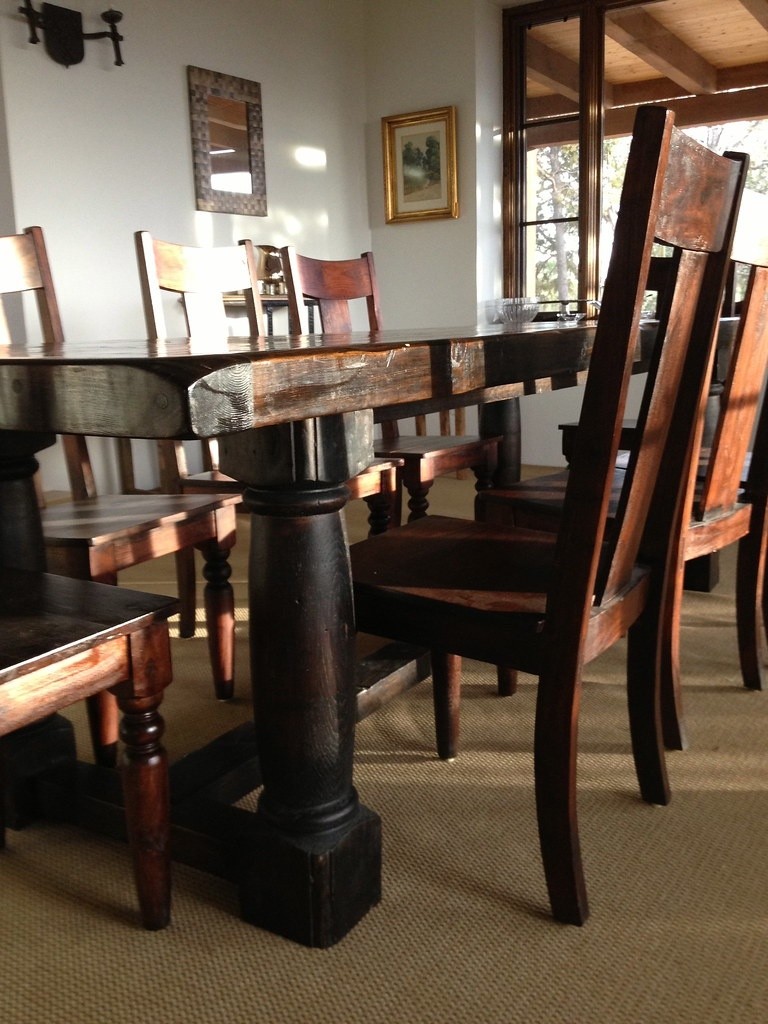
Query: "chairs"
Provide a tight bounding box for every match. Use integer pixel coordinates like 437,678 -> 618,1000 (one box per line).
0,105 -> 768,929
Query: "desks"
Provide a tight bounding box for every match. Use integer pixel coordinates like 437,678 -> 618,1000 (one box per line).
1,317 -> 741,949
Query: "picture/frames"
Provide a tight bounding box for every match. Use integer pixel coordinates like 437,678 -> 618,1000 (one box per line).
381,105 -> 458,224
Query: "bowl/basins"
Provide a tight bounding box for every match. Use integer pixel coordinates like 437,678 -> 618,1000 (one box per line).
557,313 -> 586,323
495,297 -> 540,324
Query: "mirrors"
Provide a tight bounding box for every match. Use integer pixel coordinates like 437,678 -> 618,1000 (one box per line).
187,65 -> 268,216
